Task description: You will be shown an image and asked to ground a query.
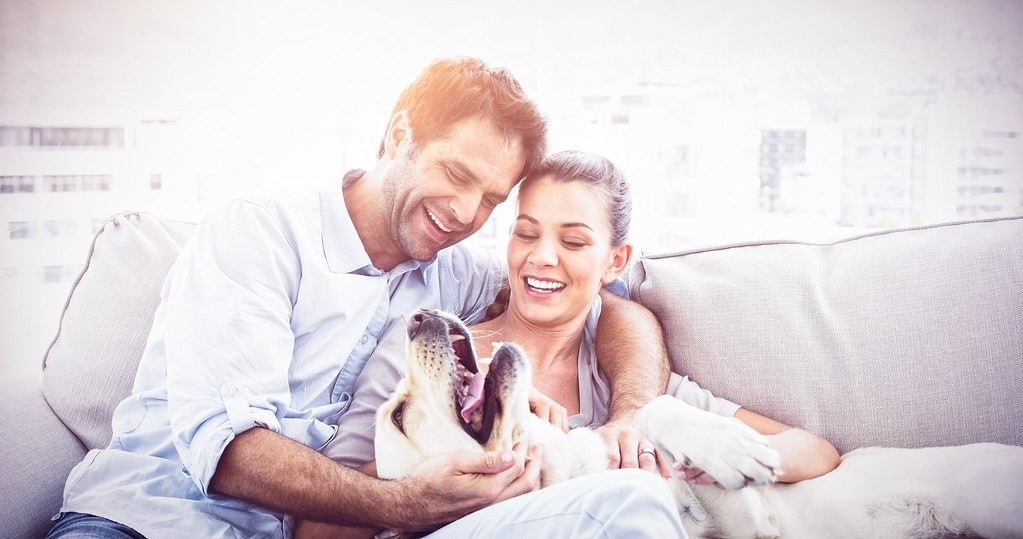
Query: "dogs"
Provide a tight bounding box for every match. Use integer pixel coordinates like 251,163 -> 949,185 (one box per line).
374,307 -> 1023,539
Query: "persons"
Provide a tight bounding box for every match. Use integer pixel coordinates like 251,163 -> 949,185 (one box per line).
42,56 -> 681,539
291,149 -> 840,539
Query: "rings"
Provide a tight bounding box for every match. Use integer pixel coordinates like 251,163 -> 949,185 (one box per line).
639,449 -> 657,457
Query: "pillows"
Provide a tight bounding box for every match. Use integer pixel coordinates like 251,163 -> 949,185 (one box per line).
629,212 -> 1023,460
41,208 -> 204,455
